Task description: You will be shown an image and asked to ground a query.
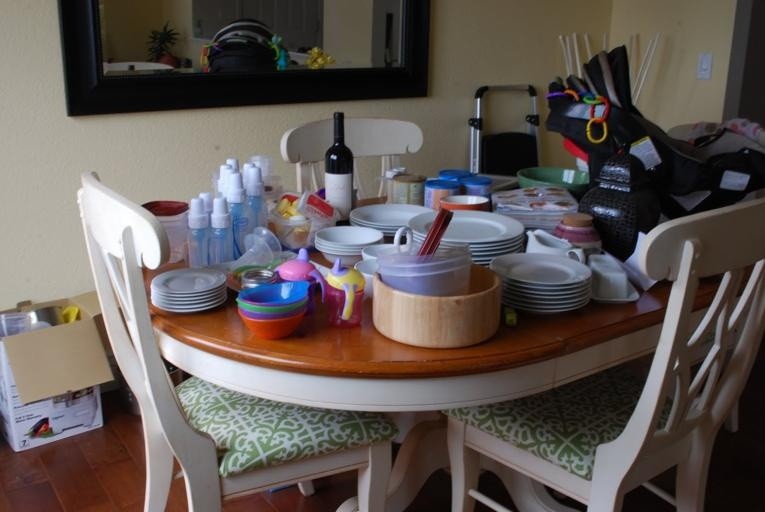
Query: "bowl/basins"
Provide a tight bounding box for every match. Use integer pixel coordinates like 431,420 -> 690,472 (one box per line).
315,225 -> 384,267
373,265 -> 503,349
236,281 -> 310,339
517,167 -> 590,196
267,198 -> 334,250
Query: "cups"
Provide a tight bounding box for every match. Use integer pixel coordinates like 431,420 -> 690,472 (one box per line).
439,195 -> 491,210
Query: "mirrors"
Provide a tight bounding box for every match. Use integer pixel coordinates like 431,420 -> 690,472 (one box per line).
56,0 -> 432,117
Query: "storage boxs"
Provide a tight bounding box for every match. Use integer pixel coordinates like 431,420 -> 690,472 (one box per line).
1,289 -> 115,453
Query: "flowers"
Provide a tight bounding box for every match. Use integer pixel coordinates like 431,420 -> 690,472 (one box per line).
305,46 -> 337,69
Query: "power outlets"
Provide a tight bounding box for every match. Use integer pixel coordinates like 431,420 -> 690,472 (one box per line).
695,49 -> 714,83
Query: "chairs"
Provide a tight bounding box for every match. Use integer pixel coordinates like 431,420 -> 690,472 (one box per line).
468,84 -> 540,177
280,118 -> 426,197
76,171 -> 401,512
442,196 -> 765,512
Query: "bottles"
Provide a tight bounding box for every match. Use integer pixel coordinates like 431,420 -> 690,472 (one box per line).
273,248 -> 365,328
324,111 -> 354,220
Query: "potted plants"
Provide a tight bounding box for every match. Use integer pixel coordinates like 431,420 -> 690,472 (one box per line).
147,19 -> 181,67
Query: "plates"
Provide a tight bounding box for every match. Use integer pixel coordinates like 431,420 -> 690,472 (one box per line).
350,204 -> 640,315
150,269 -> 228,314
